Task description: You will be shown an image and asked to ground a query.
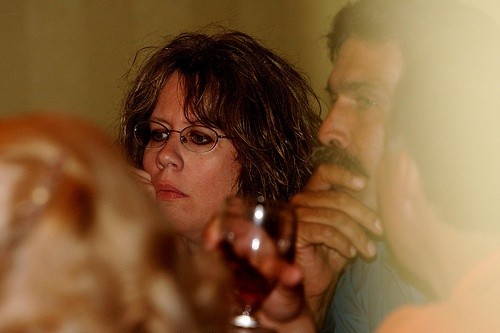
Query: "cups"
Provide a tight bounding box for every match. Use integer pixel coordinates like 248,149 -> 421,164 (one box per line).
218,191 -> 298,329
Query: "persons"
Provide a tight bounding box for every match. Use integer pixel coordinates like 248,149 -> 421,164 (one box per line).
0,107 -> 193,333
202,198 -> 319,333
371,38 -> 499,333
110,26 -> 334,333
288,1 -> 430,333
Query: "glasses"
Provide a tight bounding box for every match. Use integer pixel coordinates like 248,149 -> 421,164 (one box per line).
133,120 -> 240,154
